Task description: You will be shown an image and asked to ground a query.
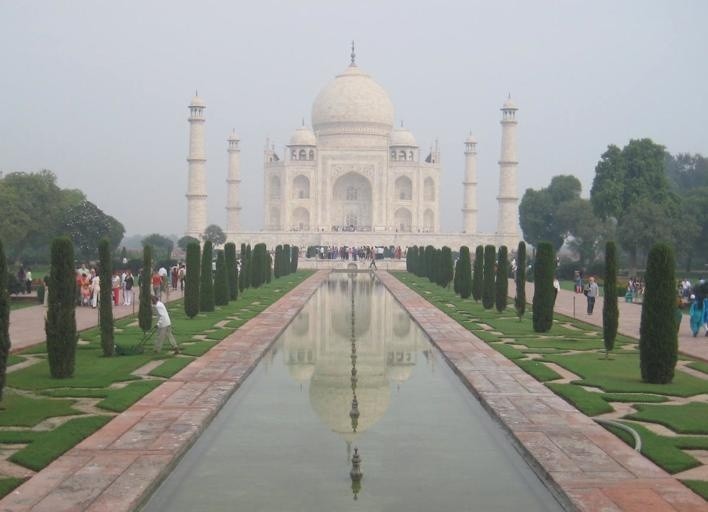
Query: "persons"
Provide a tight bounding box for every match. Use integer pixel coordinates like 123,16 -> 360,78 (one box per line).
41,276 -> 50,336
25,268 -> 32,294
494,250 -> 708,337
73,256 -> 186,309
316,244 -> 408,260
148,294 -> 181,355
368,252 -> 377,269
